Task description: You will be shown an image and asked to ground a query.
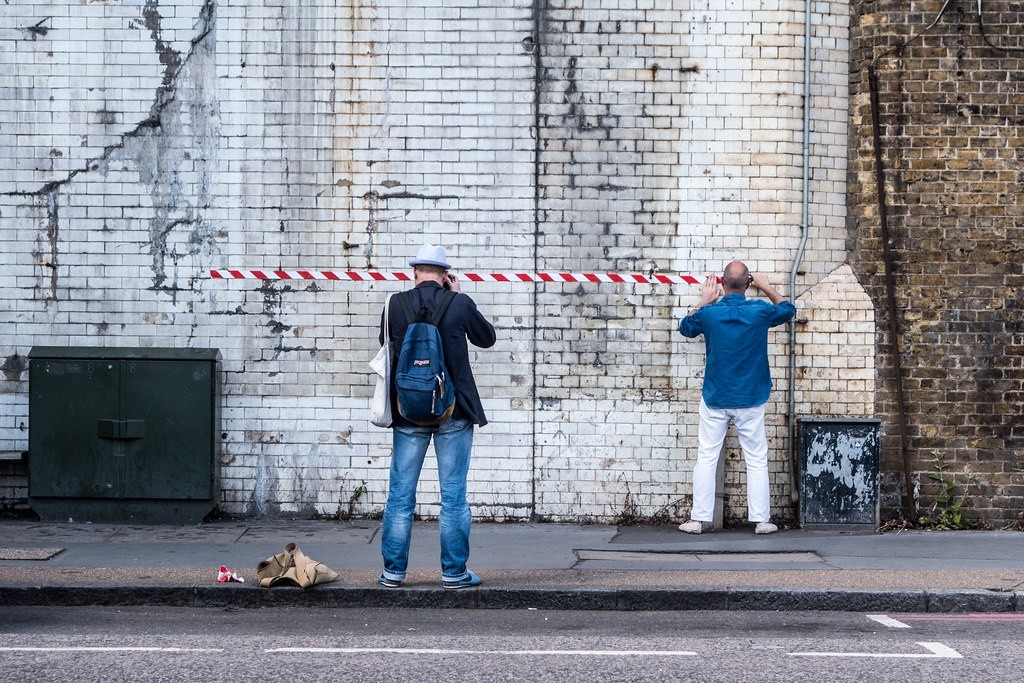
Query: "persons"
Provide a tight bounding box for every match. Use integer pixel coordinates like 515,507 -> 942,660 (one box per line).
378,243 -> 497,589
678,260 -> 797,534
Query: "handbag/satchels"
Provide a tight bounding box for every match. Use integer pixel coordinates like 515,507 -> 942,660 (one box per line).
368,343 -> 393,428
257,542 -> 339,588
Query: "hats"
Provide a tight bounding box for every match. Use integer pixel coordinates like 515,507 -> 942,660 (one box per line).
409,244 -> 452,269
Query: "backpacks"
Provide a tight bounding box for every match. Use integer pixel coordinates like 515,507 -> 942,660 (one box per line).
397,290 -> 458,427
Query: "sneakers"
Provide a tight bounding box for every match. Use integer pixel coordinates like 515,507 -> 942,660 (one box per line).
443,569 -> 482,589
678,520 -> 702,533
755,523 -> 778,534
378,574 -> 401,587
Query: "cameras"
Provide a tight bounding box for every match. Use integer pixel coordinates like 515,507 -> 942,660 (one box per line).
443,275 -> 453,290
